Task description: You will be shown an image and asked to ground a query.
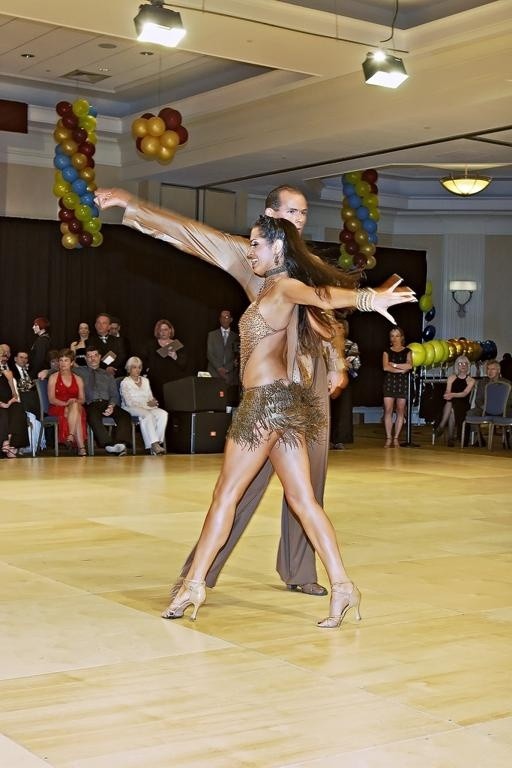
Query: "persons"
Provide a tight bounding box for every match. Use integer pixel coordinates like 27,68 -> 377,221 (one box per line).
433,356 -> 476,446
0,313 -> 188,458
160,216 -> 418,629
91,184 -> 347,595
499,353 -> 512,381
207,309 -> 240,408
467,360 -> 512,448
330,319 -> 361,450
382,327 -> 413,448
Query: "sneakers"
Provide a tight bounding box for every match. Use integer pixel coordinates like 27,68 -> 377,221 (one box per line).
104,442 -> 128,457
435,426 -> 458,448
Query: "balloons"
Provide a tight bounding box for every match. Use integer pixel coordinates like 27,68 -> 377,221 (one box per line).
338,169 -> 381,270
425,282 -> 431,294
422,326 -> 436,342
418,295 -> 433,312
425,307 -> 435,321
406,339 -> 498,367
131,108 -> 188,165
53,98 -> 103,249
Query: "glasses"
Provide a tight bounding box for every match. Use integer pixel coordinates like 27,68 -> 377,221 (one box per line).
457,362 -> 469,367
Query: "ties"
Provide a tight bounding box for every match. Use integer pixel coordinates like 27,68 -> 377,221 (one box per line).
87,369 -> 97,403
222,329 -> 228,365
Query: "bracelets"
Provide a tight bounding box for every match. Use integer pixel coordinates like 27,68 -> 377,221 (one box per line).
355,286 -> 373,312
393,363 -> 397,368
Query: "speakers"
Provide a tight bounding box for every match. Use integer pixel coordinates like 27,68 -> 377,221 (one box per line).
164,414 -> 232,454
163,377 -> 229,412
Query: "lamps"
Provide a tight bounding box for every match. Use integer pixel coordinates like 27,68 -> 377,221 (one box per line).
437,165 -> 493,201
132,0 -> 411,92
445,275 -> 480,318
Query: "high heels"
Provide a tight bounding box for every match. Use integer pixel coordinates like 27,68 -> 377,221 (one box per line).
160,576 -> 208,620
285,578 -> 328,598
317,582 -> 362,629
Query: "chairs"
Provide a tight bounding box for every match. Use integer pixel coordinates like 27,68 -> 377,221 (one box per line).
4,372 -> 170,459
427,377 -> 511,455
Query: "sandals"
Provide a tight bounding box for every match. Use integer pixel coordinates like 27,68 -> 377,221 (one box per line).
65,432 -> 74,448
384,437 -> 393,449
2,441 -> 16,458
150,444 -> 167,456
78,445 -> 88,457
392,437 -> 401,450
